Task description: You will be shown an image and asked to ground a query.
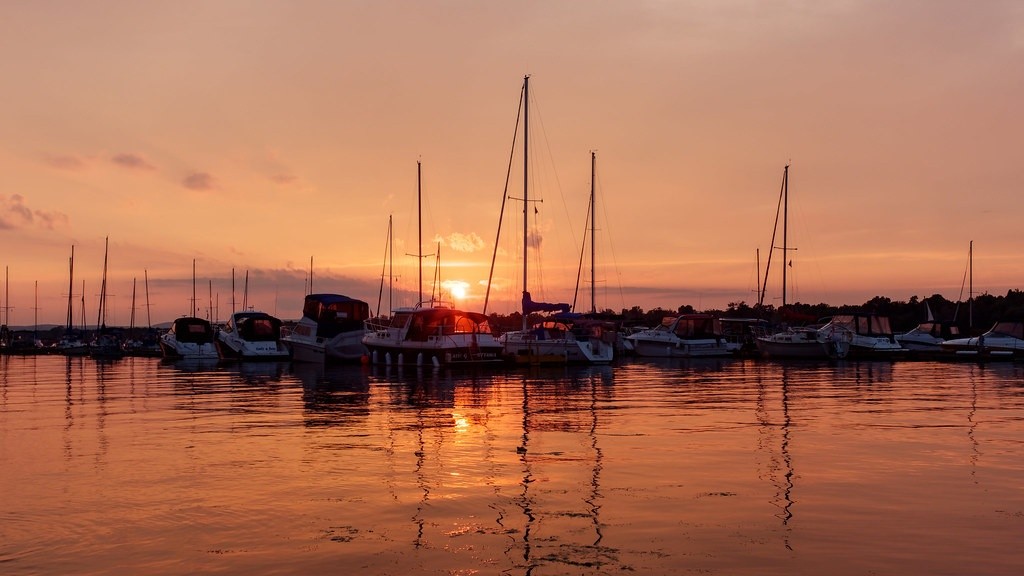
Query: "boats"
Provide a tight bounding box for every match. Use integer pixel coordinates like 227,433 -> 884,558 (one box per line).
494,321 -> 614,365
218,310 -> 290,363
623,312 -> 769,358
893,319 -> 1024,358
281,293 -> 374,362
755,314 -> 909,369
160,316 -> 220,369
362,301 -> 506,360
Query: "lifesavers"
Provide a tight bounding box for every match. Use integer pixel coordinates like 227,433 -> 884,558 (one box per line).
594,326 -> 602,338
841,330 -> 853,342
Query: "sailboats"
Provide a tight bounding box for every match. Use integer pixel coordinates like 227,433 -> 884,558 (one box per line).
0,235 -> 161,360
